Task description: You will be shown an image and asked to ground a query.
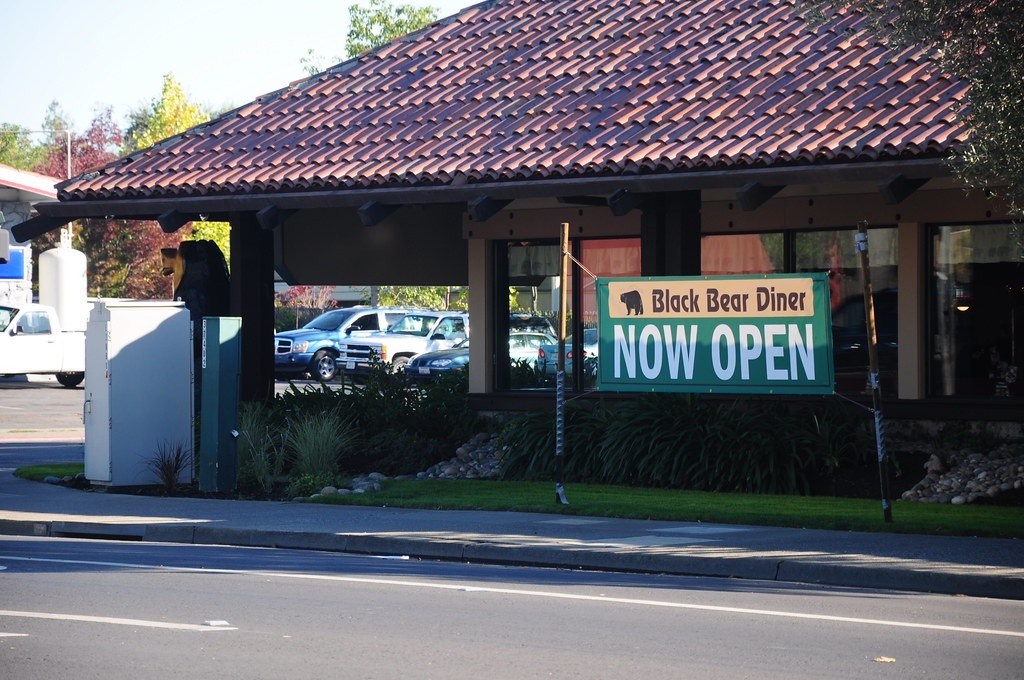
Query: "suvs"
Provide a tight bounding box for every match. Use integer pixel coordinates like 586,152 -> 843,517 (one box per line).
335,307 -> 557,387
272,308 -> 435,383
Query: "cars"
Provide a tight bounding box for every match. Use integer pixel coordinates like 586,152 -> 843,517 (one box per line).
403,333 -> 560,390
536,327 -> 599,390
829,288 -> 899,368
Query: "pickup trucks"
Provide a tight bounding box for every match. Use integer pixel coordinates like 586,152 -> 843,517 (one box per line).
0,297 -> 105,387
